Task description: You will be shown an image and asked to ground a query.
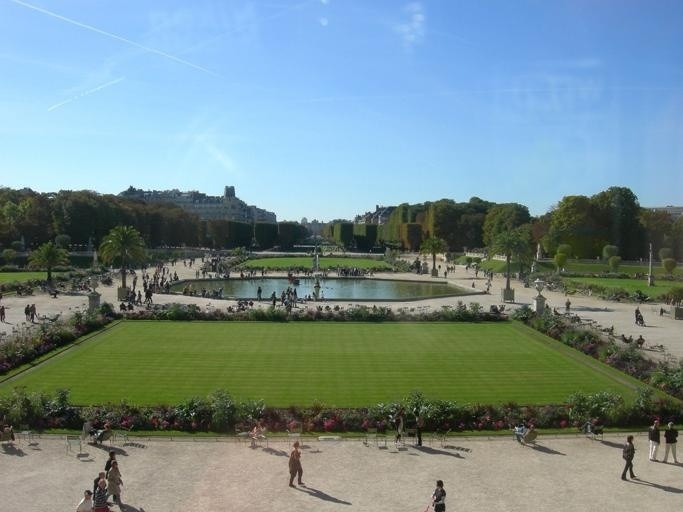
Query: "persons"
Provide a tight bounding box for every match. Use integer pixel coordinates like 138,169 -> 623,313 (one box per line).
287,440 -> 306,487
0,414 -> 15,442
393,407 -> 407,445
104,460 -> 127,510
103,451 -> 118,504
514,424 -> 535,442
75,489 -> 94,512
564,298 -> 571,312
634,306 -> 641,325
29,303 -> 37,323
620,434 -> 638,480
620,334 -> 633,344
88,417 -> 102,438
638,314 -> 644,327
412,409 -> 425,447
647,420 -> 661,462
24,304 -> 30,321
430,480 -> 446,511
119,251 -> 378,314
0,306 -> 5,322
578,414 -> 610,436
248,421 -> 265,447
660,421 -> 679,464
437,257 -> 531,293
635,334 -> 644,346
93,423 -> 111,440
90,479 -> 115,512
92,471 -> 108,505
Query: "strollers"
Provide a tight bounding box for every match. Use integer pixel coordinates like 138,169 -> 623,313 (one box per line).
636,314 -> 646,327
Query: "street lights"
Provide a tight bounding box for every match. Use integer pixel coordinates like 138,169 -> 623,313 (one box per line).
87,278 -> 102,314
532,277 -> 547,314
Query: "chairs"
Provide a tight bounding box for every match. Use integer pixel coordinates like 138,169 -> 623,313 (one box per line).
588,423 -> 605,441
95,432 -> 114,447
521,430 -> 537,449
64,435 -> 82,455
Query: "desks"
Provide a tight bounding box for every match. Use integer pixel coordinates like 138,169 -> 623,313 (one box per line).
16,430 -> 32,444
287,432 -> 303,449
